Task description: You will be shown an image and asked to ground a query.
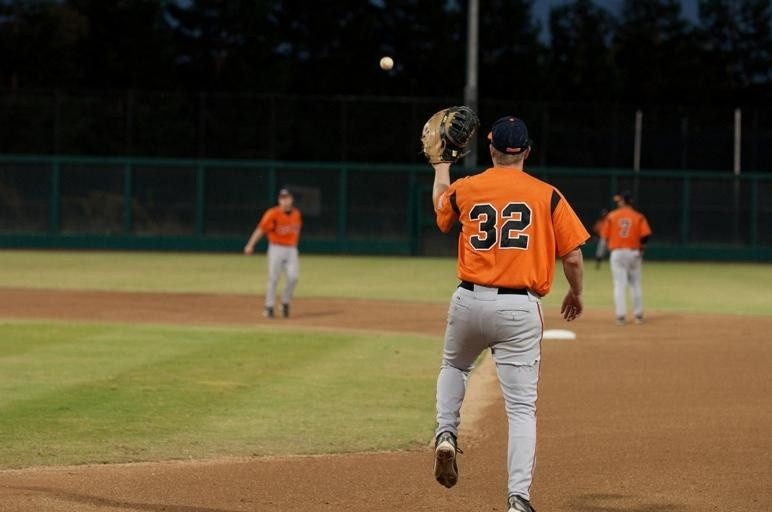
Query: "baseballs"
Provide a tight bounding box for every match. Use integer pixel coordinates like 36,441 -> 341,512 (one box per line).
380,57 -> 393,70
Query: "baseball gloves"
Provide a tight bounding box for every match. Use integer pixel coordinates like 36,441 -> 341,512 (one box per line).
421,106 -> 480,165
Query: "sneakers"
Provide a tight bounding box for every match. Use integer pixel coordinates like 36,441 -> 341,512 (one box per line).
434,433 -> 458,489
280,304 -> 289,318
507,494 -> 534,512
263,308 -> 274,318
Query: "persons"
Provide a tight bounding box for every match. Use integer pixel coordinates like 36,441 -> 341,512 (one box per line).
599,190 -> 652,326
421,105 -> 591,509
243,188 -> 303,317
592,209 -> 610,271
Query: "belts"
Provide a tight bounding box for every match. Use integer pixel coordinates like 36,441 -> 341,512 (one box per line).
457,280 -> 528,295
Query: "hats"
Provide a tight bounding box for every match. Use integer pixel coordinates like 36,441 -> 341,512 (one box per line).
614,192 -> 635,204
488,116 -> 529,154
